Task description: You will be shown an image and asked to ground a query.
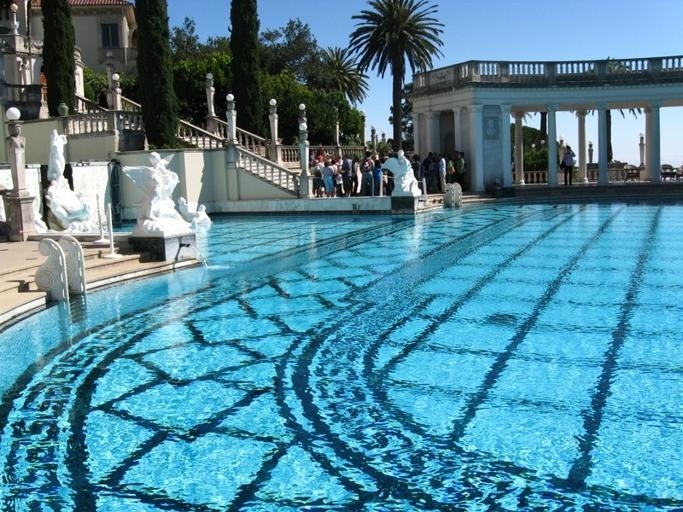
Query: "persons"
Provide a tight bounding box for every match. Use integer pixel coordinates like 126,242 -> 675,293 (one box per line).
563,146 -> 576,186
6,124 -> 26,150
309,148 -> 469,197
148,151 -> 180,184
50,129 -> 67,180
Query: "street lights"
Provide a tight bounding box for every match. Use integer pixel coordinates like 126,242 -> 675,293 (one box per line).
106,51 -> 122,112
269,98 -> 279,144
588,140 -> 593,164
359,111 -> 386,153
205,73 -> 215,116
639,132 -> 645,167
225,93 -> 237,144
4,108 -> 29,197
9,4 -> 20,35
297,103 -> 313,176
558,134 -> 565,164
